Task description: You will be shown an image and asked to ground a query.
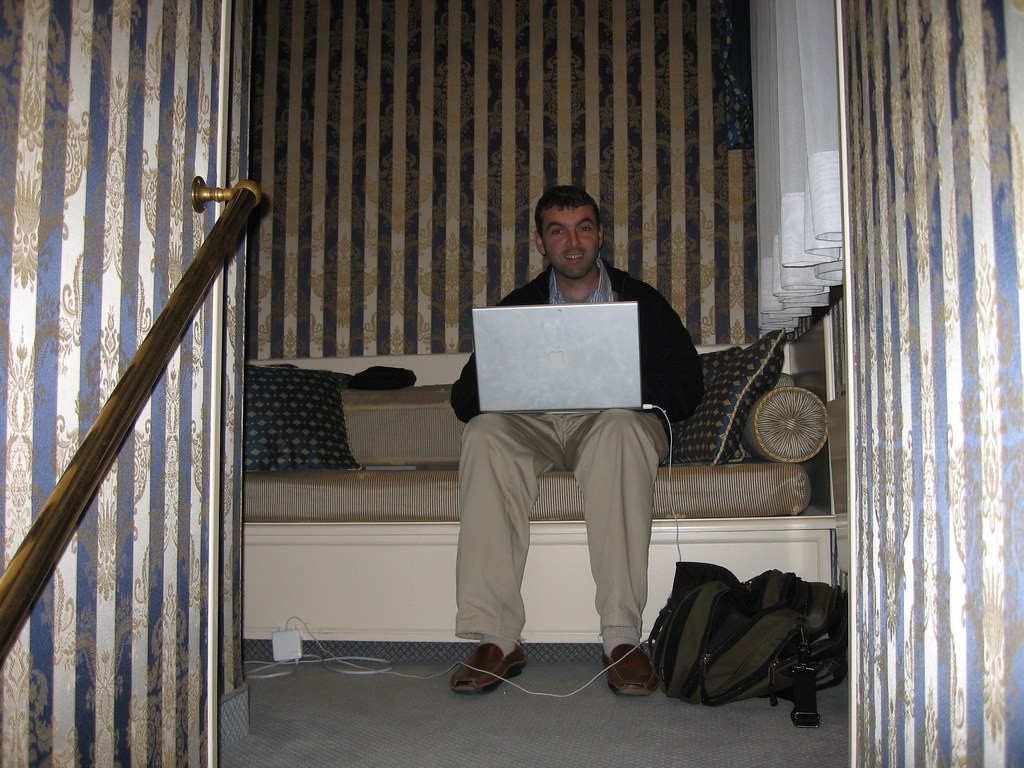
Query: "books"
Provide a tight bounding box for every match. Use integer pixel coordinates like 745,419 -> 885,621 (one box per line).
365,465 -> 417,471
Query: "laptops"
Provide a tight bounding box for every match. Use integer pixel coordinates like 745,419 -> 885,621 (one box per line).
472,301 -> 643,414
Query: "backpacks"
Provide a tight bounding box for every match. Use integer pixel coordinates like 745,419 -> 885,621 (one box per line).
652,558 -> 852,730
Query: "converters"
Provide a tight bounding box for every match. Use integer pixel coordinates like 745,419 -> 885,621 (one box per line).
272,629 -> 303,662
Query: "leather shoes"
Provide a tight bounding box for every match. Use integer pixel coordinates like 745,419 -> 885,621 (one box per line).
602,642 -> 656,695
451,636 -> 525,695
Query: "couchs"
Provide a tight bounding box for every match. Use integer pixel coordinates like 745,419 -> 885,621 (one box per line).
240,347 -> 835,665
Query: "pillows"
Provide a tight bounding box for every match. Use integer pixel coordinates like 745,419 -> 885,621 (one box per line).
663,327 -> 788,458
742,388 -> 829,464
243,363 -> 362,473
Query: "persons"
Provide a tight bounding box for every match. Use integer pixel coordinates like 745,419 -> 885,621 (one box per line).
450,185 -> 706,696
